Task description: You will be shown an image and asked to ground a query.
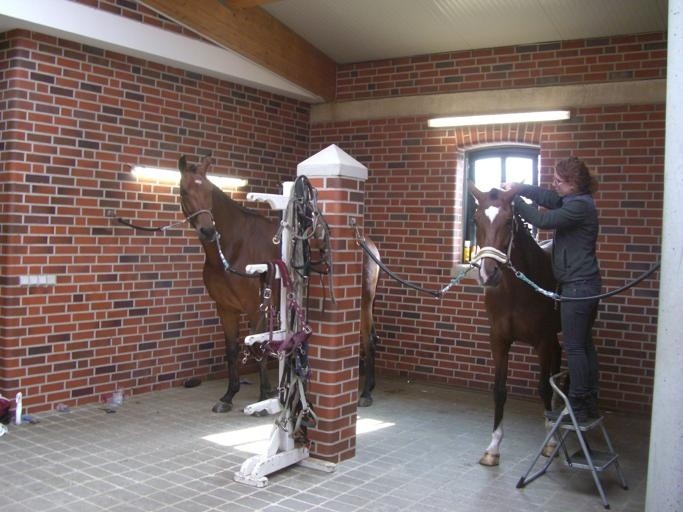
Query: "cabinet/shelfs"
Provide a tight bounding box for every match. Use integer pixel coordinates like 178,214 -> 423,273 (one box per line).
514,369 -> 629,509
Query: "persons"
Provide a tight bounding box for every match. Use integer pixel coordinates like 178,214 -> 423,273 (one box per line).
508,156 -> 602,421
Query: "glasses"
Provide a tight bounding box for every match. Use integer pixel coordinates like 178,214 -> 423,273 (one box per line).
552,176 -> 567,184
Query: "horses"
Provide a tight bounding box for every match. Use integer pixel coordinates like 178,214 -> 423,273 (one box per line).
178,155 -> 380,418
467,178 -> 563,467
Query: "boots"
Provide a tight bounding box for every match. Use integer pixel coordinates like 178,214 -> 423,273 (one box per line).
542,395 -> 601,426
